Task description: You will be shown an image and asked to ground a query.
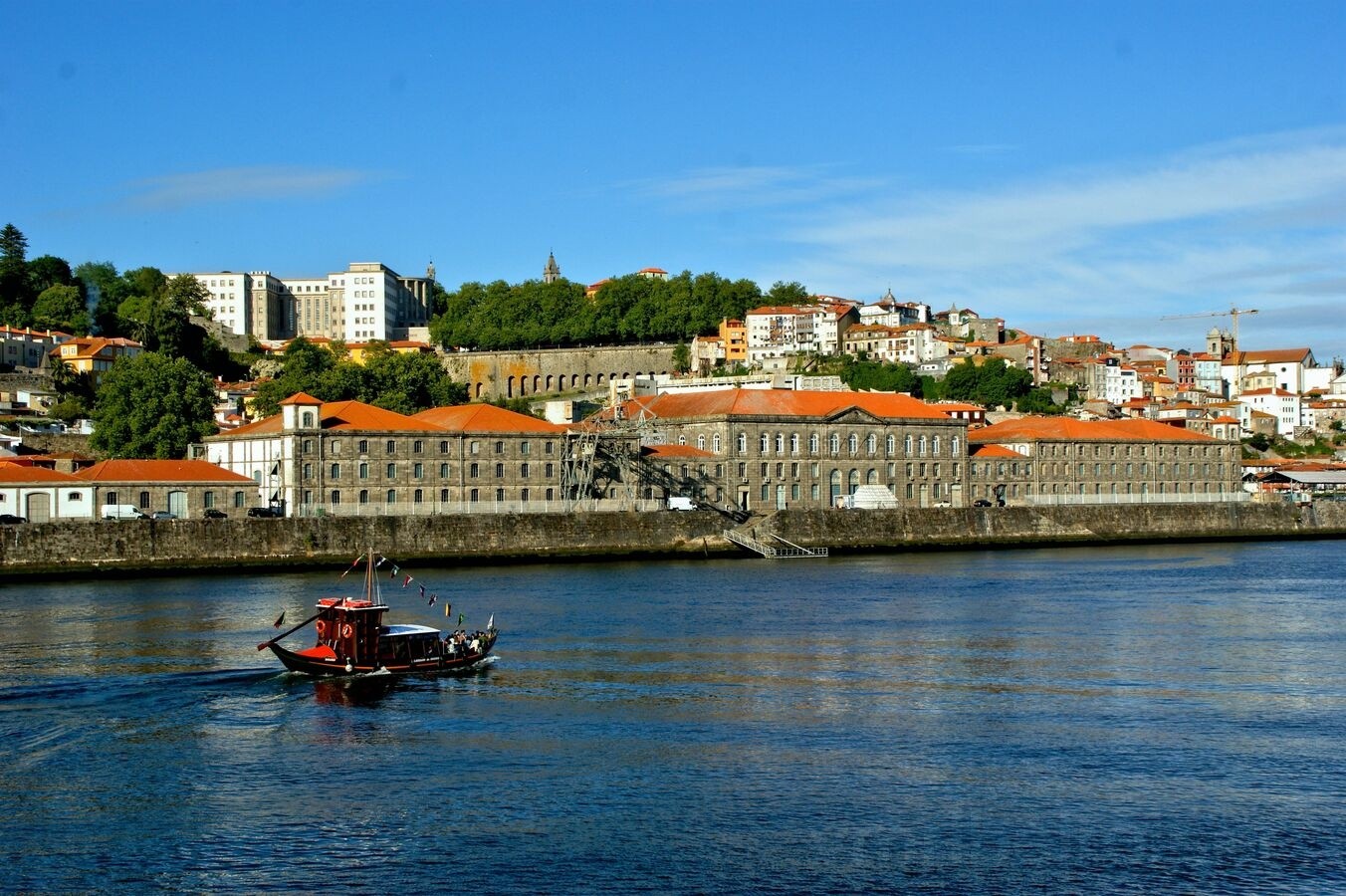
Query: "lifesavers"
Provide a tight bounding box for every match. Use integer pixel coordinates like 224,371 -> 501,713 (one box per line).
342,624 -> 352,639
316,620 -> 325,633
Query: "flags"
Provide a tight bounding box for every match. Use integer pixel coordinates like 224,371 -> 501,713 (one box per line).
420,583 -> 425,599
403,574 -> 414,588
426,593 -> 439,607
390,566 -> 400,578
445,602 -> 451,618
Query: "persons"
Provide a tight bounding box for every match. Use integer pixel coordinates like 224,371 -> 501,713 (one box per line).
443,630 -> 487,653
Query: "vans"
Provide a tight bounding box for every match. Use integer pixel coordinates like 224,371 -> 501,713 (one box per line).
101,505 -> 143,520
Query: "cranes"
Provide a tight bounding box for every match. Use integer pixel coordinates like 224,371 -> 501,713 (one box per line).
1160,303 -> 1259,394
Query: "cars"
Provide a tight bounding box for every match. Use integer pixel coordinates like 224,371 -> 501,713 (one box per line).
204,509 -> 228,519
153,511 -> 178,519
974,500 -> 992,507
0,514 -> 27,525
934,501 -> 952,508
248,508 -> 277,518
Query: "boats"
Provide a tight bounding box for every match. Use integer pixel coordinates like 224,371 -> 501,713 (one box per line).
258,545 -> 499,677
